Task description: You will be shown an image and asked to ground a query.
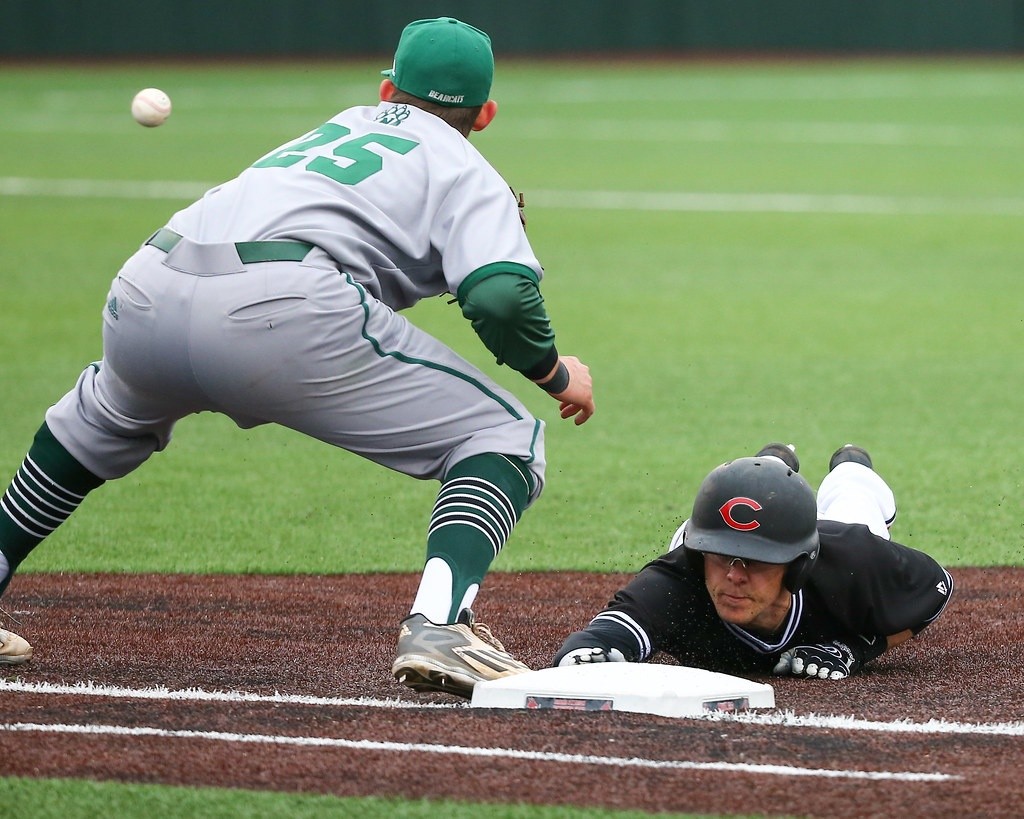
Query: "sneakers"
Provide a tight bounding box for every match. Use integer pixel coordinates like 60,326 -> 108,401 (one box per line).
392,606 -> 533,702
0,627 -> 33,666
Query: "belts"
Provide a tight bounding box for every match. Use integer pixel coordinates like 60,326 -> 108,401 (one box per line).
147,227 -> 310,264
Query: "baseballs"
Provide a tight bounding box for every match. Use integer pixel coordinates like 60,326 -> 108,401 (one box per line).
130,88 -> 174,126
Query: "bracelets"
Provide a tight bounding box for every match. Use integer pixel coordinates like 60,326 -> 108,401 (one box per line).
536,359 -> 570,395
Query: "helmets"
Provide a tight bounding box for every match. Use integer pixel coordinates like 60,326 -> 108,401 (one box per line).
681,451 -> 818,592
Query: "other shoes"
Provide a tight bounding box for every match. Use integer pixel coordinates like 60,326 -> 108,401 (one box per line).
825,444 -> 874,473
753,444 -> 800,473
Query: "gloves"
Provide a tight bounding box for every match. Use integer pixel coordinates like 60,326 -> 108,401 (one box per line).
771,641 -> 856,681
559,643 -> 627,664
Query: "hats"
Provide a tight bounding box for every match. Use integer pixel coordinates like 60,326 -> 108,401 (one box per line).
381,16 -> 494,106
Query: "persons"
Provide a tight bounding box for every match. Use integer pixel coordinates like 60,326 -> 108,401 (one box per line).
553,444 -> 953,683
1,16 -> 595,705
